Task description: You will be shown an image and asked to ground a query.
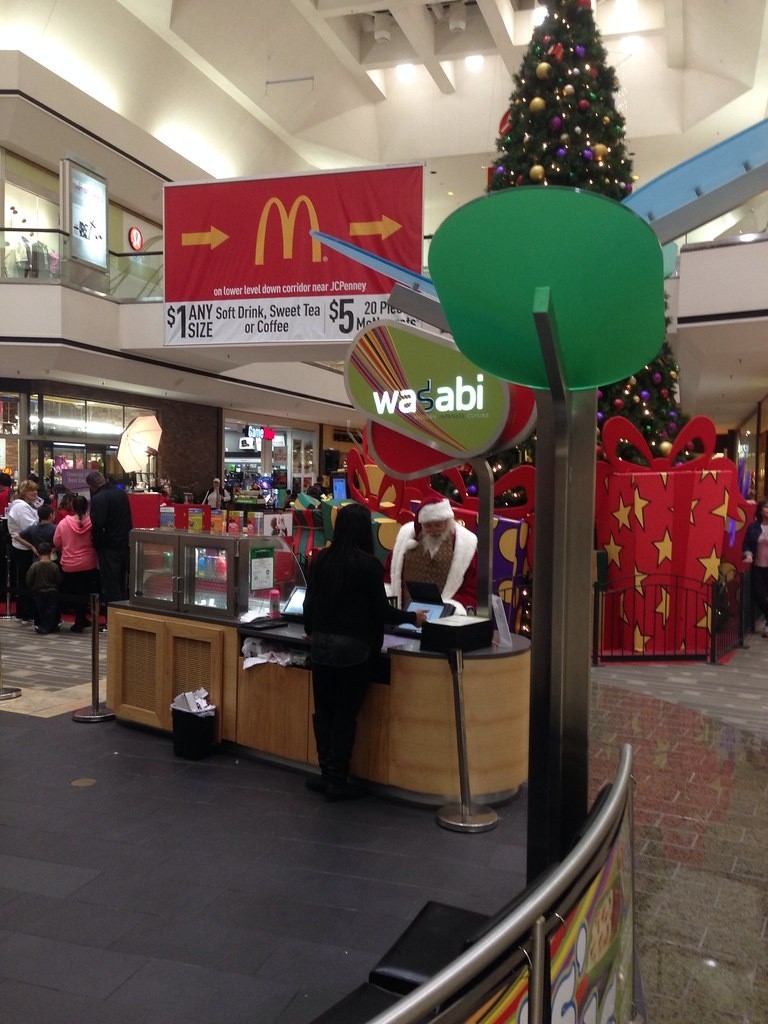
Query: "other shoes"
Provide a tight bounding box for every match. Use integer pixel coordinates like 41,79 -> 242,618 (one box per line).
14,616 -> 22,623
57,619 -> 64,632
35,625 -> 39,633
306,775 -> 330,792
324,781 -> 368,801
22,619 -> 34,624
98,623 -> 108,634
761,626 -> 768,636
70,616 -> 91,634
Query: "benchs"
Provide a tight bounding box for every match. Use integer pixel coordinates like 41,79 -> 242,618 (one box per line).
307,743 -> 638,1024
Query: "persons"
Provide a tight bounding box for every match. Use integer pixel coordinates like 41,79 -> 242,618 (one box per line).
250,482 -> 264,499
283,489 -> 298,511
383,496 -> 479,616
53,495 -> 107,631
302,504 -> 429,805
85,471 -> 134,633
742,500 -> 768,639
201,479 -> 231,510
0,468 -> 77,633
12,232 -> 59,279
306,476 -> 329,500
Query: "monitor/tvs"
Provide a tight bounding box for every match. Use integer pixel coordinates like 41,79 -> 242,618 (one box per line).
330,471 -> 353,498
281,585 -> 306,622
394,598 -> 457,640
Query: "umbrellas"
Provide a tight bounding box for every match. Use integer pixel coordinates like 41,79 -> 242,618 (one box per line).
116,416 -> 163,474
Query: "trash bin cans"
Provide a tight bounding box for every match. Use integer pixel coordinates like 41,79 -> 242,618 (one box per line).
172,703 -> 218,762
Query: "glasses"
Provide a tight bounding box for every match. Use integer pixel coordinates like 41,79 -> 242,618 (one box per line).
214,481 -> 218,483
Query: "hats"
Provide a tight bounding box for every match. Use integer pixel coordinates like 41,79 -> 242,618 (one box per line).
213,477 -> 220,483
404,495 -> 454,550
